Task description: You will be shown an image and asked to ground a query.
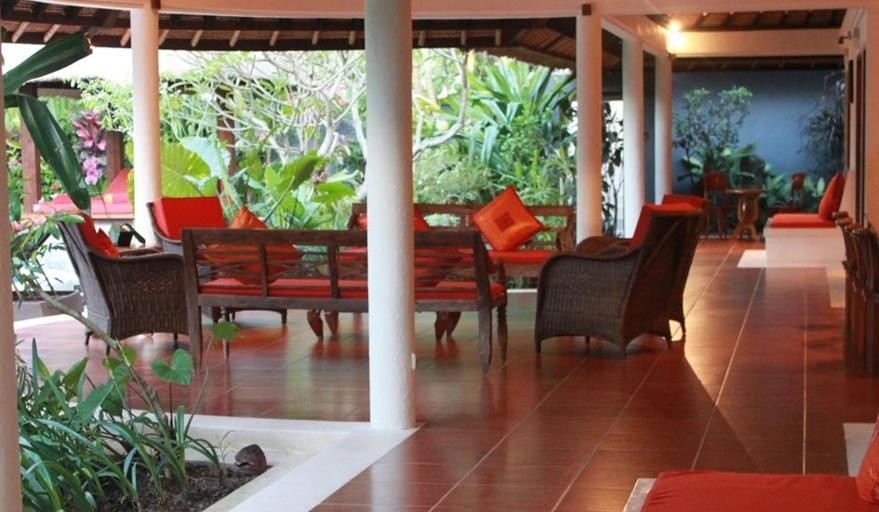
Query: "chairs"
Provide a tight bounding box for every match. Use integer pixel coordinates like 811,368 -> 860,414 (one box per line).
146,196 -> 288,358
535,195 -> 700,362
760,170 -> 858,266
56,212 -> 190,357
701,171 -> 743,241
766,172 -> 806,218
638,419 -> 879,511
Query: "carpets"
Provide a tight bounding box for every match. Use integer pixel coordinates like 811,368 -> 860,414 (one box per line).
841,420 -> 875,477
738,248 -> 767,268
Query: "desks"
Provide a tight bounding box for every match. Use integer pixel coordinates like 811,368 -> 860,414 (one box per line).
724,187 -> 770,241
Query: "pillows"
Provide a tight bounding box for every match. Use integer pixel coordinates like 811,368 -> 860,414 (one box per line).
200,207 -> 303,287
470,185 -> 544,251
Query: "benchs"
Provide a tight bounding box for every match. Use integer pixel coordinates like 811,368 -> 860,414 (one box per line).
345,201 -> 574,336
181,225 -> 508,373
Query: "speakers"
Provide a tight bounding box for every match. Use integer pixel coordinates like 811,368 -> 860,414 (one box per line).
582,4 -> 591,15
152,0 -> 161,9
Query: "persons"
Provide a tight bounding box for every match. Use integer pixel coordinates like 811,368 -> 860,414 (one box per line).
773,171 -> 815,208
